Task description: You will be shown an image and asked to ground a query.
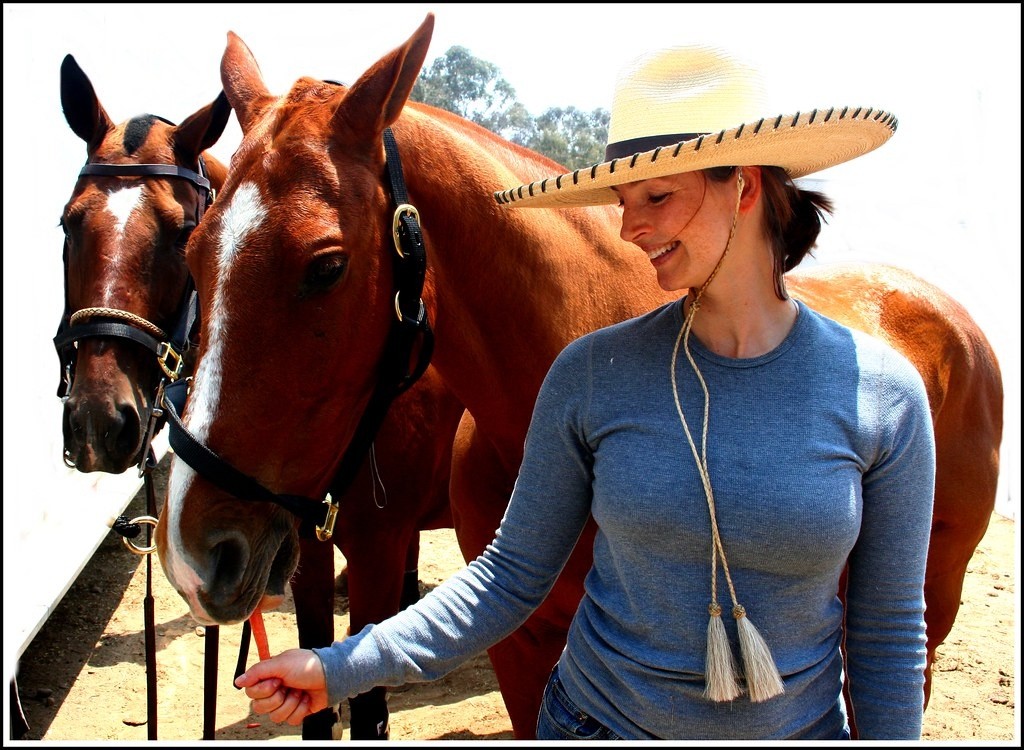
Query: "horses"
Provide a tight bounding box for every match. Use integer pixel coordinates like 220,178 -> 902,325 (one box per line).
54,13 -> 1004,740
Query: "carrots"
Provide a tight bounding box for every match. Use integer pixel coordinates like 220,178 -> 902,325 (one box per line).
248,606 -> 270,660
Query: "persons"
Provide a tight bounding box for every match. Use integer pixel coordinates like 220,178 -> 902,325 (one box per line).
234,46 -> 939,740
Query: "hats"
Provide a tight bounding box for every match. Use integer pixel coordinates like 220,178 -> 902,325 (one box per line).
494,42 -> 898,209
